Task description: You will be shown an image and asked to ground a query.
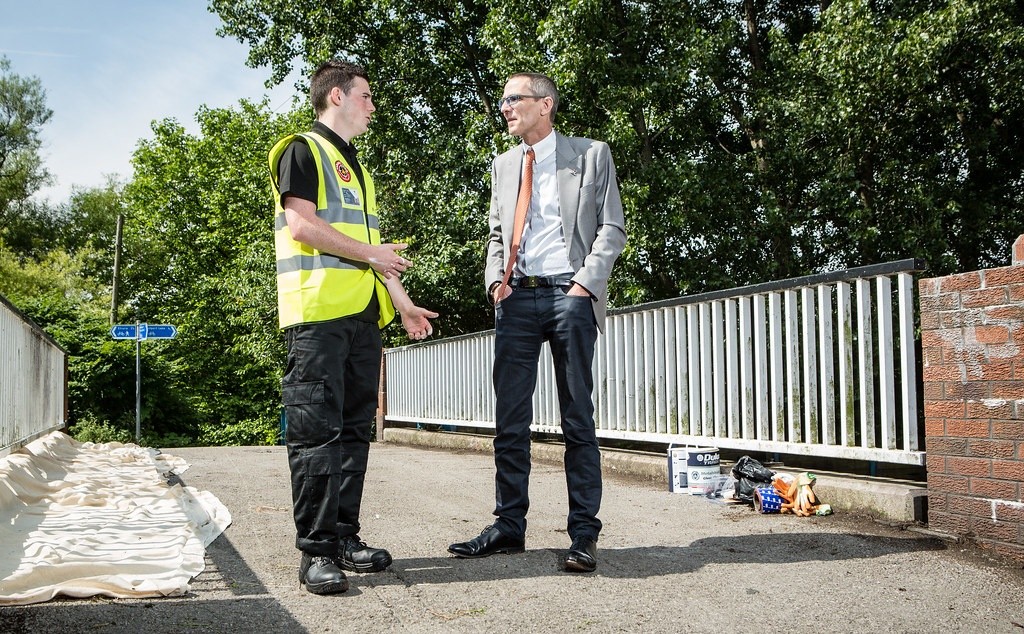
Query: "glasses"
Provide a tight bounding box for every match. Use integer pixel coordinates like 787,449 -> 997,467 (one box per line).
498,95 -> 546,110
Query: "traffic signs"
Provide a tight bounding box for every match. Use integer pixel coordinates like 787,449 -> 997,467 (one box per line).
111,324 -> 175,341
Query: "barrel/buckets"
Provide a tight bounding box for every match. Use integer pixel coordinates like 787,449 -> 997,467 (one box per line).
687,444 -> 720,496
667,444 -> 689,494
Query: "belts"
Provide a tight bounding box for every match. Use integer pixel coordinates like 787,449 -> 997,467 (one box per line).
506,276 -> 574,287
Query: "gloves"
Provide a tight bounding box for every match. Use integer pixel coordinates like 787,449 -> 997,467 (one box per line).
772,472 -> 832,517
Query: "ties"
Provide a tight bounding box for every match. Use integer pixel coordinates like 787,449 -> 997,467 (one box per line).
495,150 -> 535,303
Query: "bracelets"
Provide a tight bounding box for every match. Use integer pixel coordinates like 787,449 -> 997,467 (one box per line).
489,282 -> 499,293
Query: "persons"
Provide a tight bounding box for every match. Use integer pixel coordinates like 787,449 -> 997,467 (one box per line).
448,72 -> 627,572
268,60 -> 440,594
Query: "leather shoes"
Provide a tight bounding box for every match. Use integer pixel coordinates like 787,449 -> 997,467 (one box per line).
565,535 -> 597,571
446,525 -> 525,558
298,551 -> 348,595
330,537 -> 392,573
